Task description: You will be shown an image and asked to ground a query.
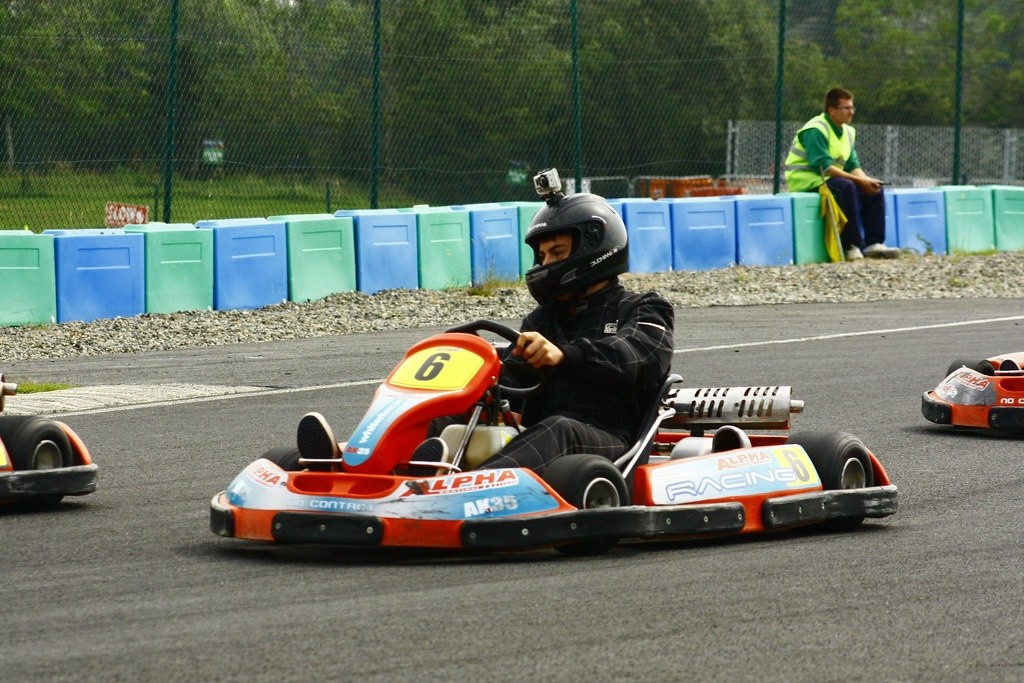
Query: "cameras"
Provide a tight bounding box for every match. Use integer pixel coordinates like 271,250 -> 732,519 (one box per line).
533,168 -> 562,195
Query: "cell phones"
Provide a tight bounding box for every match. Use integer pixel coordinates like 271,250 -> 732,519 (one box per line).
879,183 -> 891,185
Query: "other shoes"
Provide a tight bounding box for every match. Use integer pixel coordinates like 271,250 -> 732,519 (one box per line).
861,243 -> 900,260
410,436 -> 450,478
845,244 -> 864,261
296,411 -> 344,471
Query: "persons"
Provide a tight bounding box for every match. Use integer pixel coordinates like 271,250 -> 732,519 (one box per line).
294,190 -> 675,479
785,87 -> 900,261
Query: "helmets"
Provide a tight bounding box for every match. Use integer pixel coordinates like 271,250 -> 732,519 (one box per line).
524,192 -> 629,305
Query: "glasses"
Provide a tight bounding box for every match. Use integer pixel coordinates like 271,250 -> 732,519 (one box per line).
836,106 -> 855,112
